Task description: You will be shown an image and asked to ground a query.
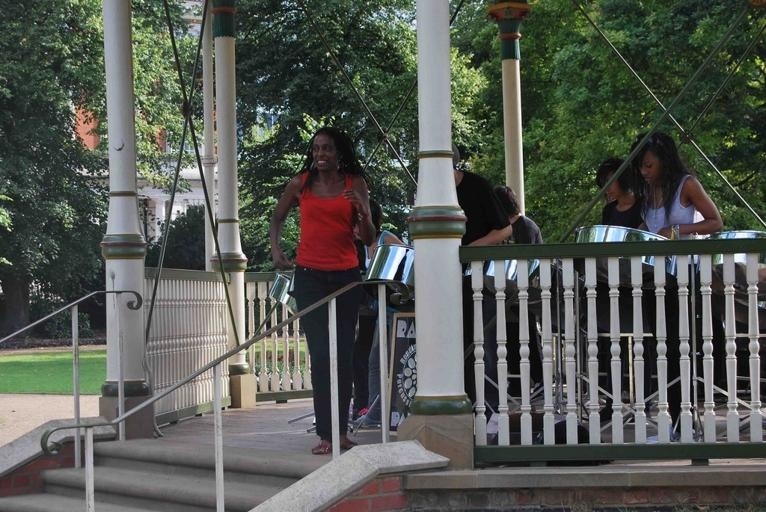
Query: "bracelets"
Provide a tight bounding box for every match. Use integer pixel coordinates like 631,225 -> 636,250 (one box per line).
671,224 -> 681,240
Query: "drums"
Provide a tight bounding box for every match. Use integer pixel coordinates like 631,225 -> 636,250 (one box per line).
711,230 -> 766,297
573,224 -> 677,289
464,259 -> 542,304
269,272 -> 298,315
365,245 -> 415,313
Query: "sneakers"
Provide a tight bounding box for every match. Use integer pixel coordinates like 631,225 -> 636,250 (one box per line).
355,407 -> 381,427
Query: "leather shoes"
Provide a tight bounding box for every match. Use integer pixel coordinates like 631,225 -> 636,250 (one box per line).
340,436 -> 357,450
312,440 -> 331,455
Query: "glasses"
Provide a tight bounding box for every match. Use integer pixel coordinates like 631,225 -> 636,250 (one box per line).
635,133 -> 663,146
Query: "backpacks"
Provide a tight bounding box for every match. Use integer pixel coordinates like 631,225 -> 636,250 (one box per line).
353,213 -> 368,273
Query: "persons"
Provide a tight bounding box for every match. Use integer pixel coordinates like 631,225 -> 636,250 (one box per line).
448,143 -> 514,422
268,125 -> 379,455
594,158 -> 652,230
343,199 -> 407,429
627,128 -> 724,446
492,183 -> 553,406
